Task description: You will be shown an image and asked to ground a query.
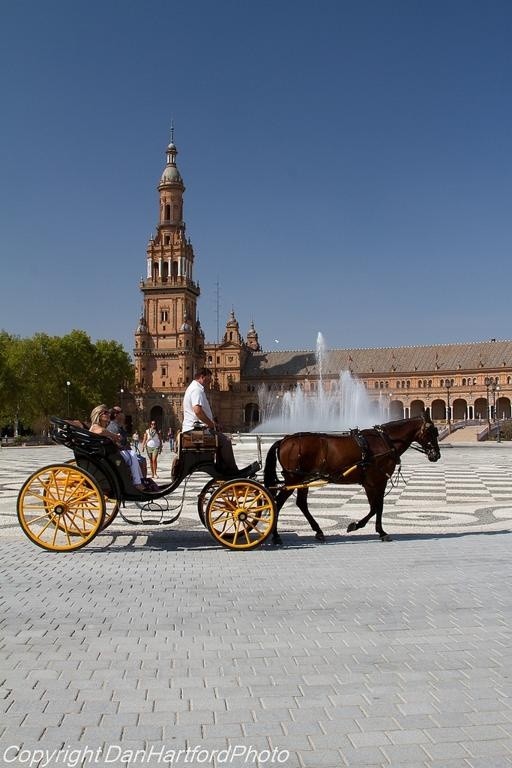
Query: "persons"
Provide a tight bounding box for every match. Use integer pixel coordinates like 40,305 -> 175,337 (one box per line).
106,406 -> 147,479
133,430 -> 140,452
141,419 -> 161,478
159,426 -> 181,453
88,404 -> 149,492
183,368 -> 245,476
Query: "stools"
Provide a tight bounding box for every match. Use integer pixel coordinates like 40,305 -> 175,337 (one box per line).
177,431 -> 215,448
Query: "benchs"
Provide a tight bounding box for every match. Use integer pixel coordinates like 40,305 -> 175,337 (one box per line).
65,419 -> 147,479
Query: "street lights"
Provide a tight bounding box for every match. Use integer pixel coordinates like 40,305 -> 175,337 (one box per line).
492,383 -> 496,422
445,380 -> 451,424
484,378 -> 490,423
67,381 -> 71,416
496,385 -> 501,443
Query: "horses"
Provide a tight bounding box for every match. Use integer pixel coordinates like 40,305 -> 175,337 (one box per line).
264,407 -> 441,545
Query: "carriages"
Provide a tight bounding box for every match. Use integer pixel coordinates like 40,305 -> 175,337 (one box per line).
17,408 -> 440,552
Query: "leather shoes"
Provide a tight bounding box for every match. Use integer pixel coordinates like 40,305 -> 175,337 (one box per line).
134,484 -> 146,491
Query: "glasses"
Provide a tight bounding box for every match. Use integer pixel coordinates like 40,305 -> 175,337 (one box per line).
118,409 -> 123,413
99,410 -> 110,418
